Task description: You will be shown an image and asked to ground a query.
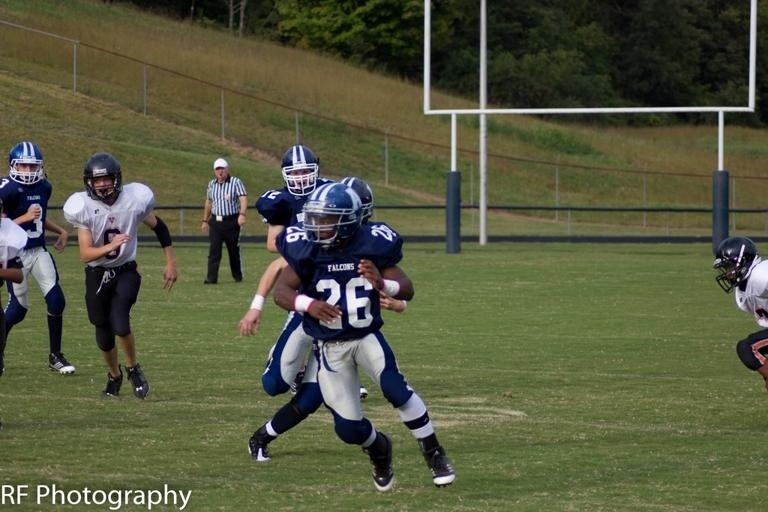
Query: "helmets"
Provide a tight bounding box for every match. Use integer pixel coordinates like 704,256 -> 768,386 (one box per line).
713,235 -> 758,294
304,184 -> 364,248
82,153 -> 122,200
340,176 -> 374,221
281,144 -> 320,197
8,141 -> 44,185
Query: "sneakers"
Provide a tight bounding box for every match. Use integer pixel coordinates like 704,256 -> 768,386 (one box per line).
125,365 -> 150,399
370,432 -> 394,492
248,435 -> 271,465
423,446 -> 456,488
48,353 -> 76,374
105,364 -> 123,400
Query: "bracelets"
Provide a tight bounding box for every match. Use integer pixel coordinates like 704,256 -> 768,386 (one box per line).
240,213 -> 246,217
202,220 -> 208,223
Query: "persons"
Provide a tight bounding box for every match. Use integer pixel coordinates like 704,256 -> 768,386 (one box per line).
715,235 -> 768,382
63,152 -> 177,398
1,201 -> 27,374
0,141 -> 74,375
201,158 -> 247,283
240,143 -> 455,489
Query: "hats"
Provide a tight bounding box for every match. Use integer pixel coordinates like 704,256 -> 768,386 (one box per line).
213,158 -> 229,171
